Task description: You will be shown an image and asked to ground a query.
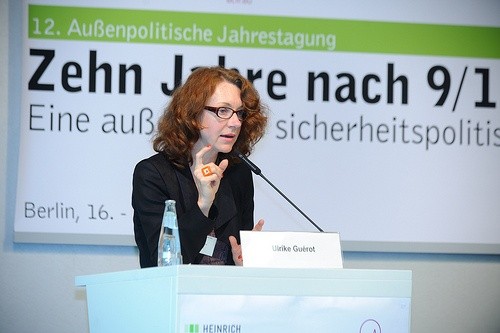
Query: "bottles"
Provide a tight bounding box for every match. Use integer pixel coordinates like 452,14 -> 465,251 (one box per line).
158,199 -> 182,266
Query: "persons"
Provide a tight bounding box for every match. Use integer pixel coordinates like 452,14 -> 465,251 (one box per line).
132,66 -> 268,268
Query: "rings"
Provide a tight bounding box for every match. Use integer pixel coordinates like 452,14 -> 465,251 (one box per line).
201,165 -> 212,176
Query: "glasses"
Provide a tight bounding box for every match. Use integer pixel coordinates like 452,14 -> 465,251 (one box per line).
203,105 -> 248,121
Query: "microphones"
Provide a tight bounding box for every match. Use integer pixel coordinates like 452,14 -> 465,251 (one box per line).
234,149 -> 261,175
